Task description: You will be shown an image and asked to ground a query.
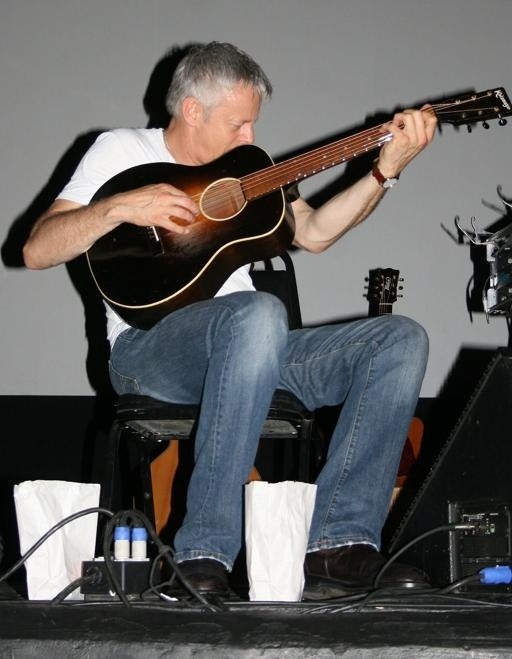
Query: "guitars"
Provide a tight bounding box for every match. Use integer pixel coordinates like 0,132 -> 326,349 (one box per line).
363,268 -> 424,512
83,86 -> 512,330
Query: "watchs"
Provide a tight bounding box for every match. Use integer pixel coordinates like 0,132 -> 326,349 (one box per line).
371,156 -> 401,189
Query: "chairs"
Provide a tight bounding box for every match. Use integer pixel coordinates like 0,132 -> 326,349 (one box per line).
94,250 -> 317,555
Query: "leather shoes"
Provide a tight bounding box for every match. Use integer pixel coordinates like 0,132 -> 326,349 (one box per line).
166,559 -> 231,602
302,545 -> 431,601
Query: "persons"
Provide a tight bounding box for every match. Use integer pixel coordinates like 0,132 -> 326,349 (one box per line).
23,40 -> 439,601
